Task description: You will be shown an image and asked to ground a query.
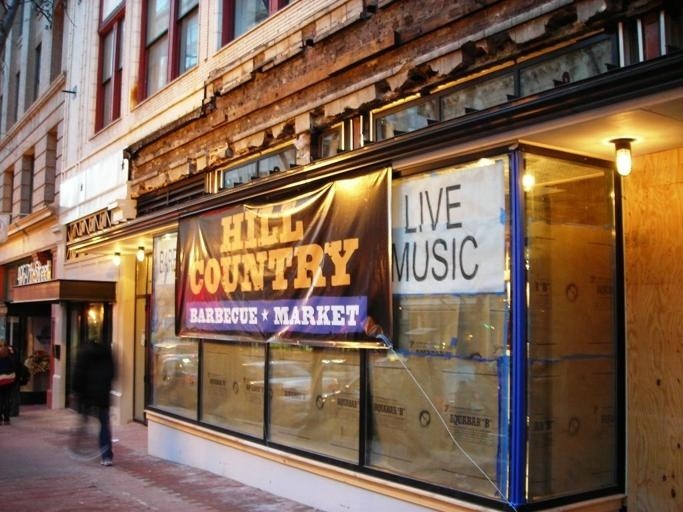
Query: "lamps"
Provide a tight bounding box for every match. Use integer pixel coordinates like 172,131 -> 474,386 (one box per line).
609,139 -> 636,177
112,252 -> 120,266
136,246 -> 145,262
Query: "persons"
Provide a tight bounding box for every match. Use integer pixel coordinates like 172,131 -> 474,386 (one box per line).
0,339 -> 28,425
64,323 -> 115,466
